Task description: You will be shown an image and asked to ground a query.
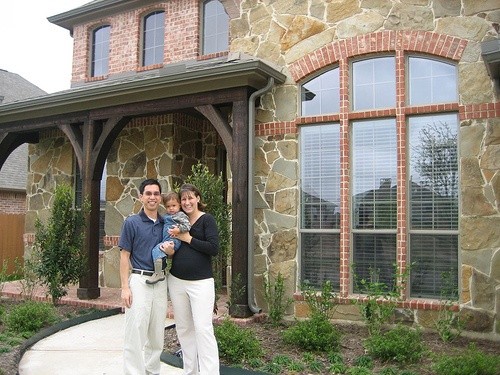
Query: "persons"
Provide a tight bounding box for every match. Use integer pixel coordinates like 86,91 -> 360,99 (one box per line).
167,183 -> 220,375
145,191 -> 191,284
118,179 -> 167,375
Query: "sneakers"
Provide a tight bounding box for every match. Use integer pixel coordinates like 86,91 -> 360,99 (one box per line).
146,272 -> 165,284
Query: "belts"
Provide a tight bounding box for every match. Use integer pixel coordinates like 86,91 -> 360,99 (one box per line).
131,269 -> 165,276
